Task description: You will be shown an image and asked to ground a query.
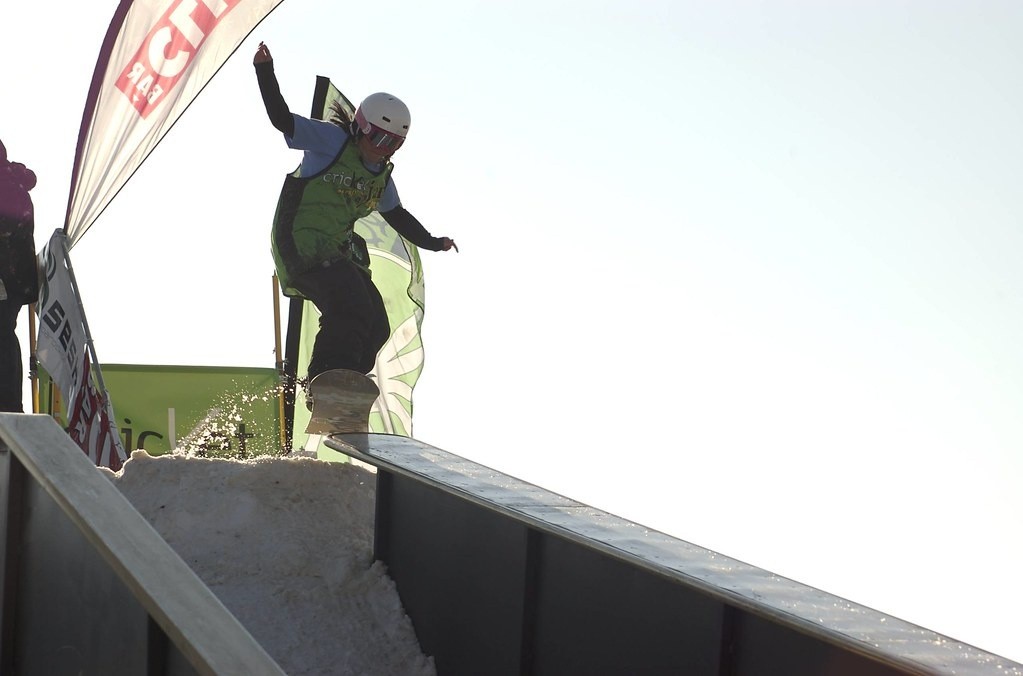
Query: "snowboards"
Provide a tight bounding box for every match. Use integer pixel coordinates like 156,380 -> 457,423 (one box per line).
304,368 -> 383,436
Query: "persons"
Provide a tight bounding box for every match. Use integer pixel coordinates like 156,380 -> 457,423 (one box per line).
0,142 -> 38,453
254,42 -> 459,409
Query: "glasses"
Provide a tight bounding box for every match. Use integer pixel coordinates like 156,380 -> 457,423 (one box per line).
363,122 -> 405,156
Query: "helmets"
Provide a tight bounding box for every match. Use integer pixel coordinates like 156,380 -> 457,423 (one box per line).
353,92 -> 411,139
